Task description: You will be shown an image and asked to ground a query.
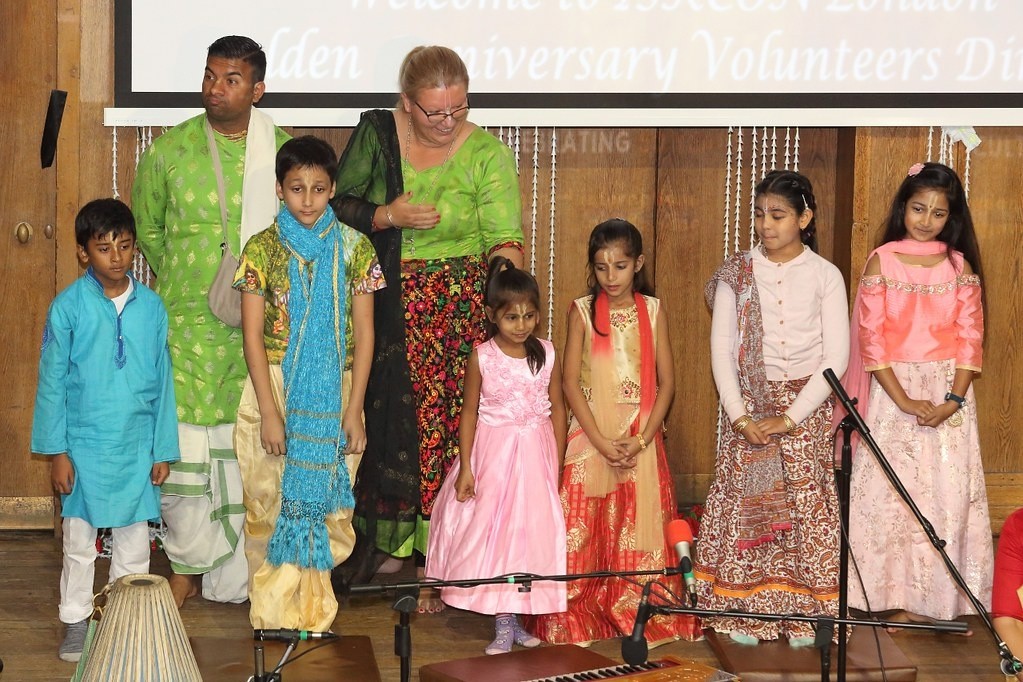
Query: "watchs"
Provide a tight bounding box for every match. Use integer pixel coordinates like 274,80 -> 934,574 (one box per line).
944,392 -> 968,408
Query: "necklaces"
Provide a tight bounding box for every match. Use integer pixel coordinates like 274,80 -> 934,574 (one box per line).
212,128 -> 248,143
401,114 -> 458,256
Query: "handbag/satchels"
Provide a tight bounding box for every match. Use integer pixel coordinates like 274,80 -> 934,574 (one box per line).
208,243 -> 242,328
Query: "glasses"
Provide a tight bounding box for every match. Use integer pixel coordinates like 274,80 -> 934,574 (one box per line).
415,94 -> 470,123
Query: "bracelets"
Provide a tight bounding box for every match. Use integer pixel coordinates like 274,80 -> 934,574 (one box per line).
636,433 -> 647,452
371,220 -> 383,231
732,416 -> 749,433
780,413 -> 793,431
386,206 -> 398,229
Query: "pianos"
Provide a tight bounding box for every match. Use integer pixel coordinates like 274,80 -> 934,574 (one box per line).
523,653 -> 684,681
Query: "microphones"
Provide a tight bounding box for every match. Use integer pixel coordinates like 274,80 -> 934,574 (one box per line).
621,583 -> 651,666
667,519 -> 698,601
1000,659 -> 1023,676
254,629 -> 339,641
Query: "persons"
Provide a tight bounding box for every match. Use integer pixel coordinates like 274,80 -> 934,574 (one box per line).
425,257 -> 566,655
992,507 -> 1023,682
130,36 -> 295,609
686,169 -> 857,648
831,161 -> 994,635
328,46 -> 524,612
31,197 -> 183,664
526,218 -> 704,647
232,135 -> 387,633
